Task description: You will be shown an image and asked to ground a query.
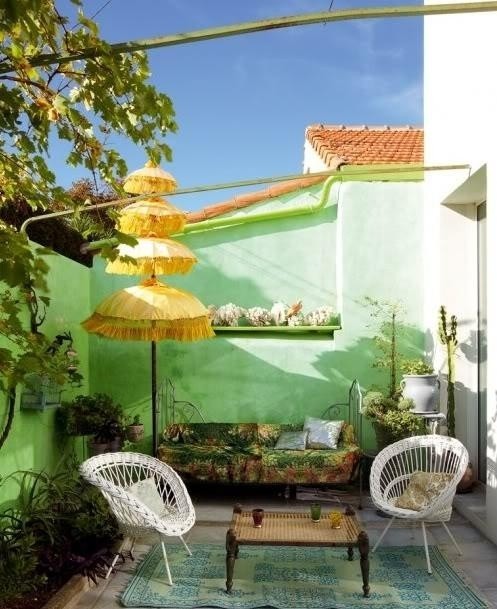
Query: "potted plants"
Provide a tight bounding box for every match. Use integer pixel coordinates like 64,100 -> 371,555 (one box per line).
56,392 -> 144,459
359,297 -> 473,494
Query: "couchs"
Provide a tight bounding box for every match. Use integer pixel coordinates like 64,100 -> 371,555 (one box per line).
154,377 -> 363,509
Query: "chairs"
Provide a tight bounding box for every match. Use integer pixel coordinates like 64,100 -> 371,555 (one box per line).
78,452 -> 196,586
369,435 -> 469,573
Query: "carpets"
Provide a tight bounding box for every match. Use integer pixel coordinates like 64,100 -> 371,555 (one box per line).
119,543 -> 496,609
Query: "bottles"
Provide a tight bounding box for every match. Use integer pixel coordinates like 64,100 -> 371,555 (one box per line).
284,485 -> 291,500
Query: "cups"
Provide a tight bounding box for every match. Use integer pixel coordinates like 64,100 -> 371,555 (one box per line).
252,509 -> 263,529
311,504 -> 321,523
329,509 -> 341,529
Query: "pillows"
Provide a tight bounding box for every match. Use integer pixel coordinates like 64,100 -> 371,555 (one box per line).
272,415 -> 345,451
124,476 -> 170,519
394,470 -> 455,512
